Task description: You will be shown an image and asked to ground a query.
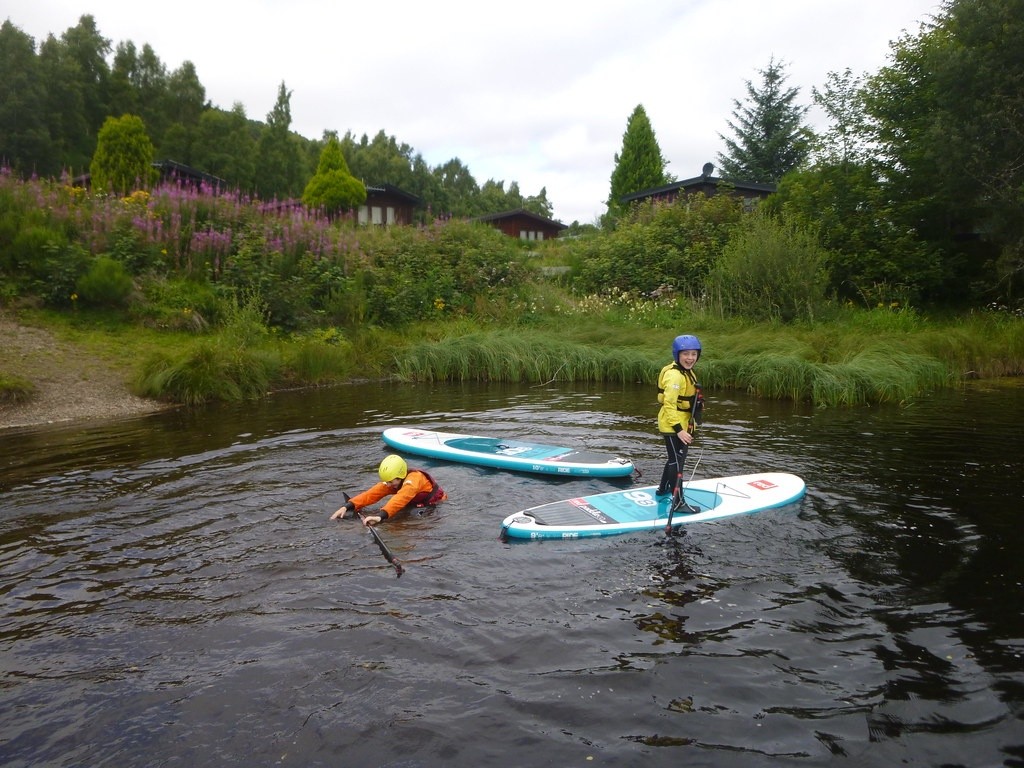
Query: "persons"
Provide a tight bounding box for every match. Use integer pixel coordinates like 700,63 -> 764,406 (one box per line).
657,335 -> 703,516
329,455 -> 446,525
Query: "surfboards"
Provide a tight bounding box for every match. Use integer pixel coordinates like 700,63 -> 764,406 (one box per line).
500,472 -> 806,539
383,427 -> 633,478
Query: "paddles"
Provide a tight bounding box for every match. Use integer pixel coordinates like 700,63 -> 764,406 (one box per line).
667,384 -> 701,532
342,492 -> 402,571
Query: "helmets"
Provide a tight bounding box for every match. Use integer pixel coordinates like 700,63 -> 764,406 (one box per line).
672,335 -> 700,366
379,454 -> 408,482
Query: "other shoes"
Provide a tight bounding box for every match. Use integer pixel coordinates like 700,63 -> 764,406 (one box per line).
654,486 -> 673,495
673,503 -> 702,513
